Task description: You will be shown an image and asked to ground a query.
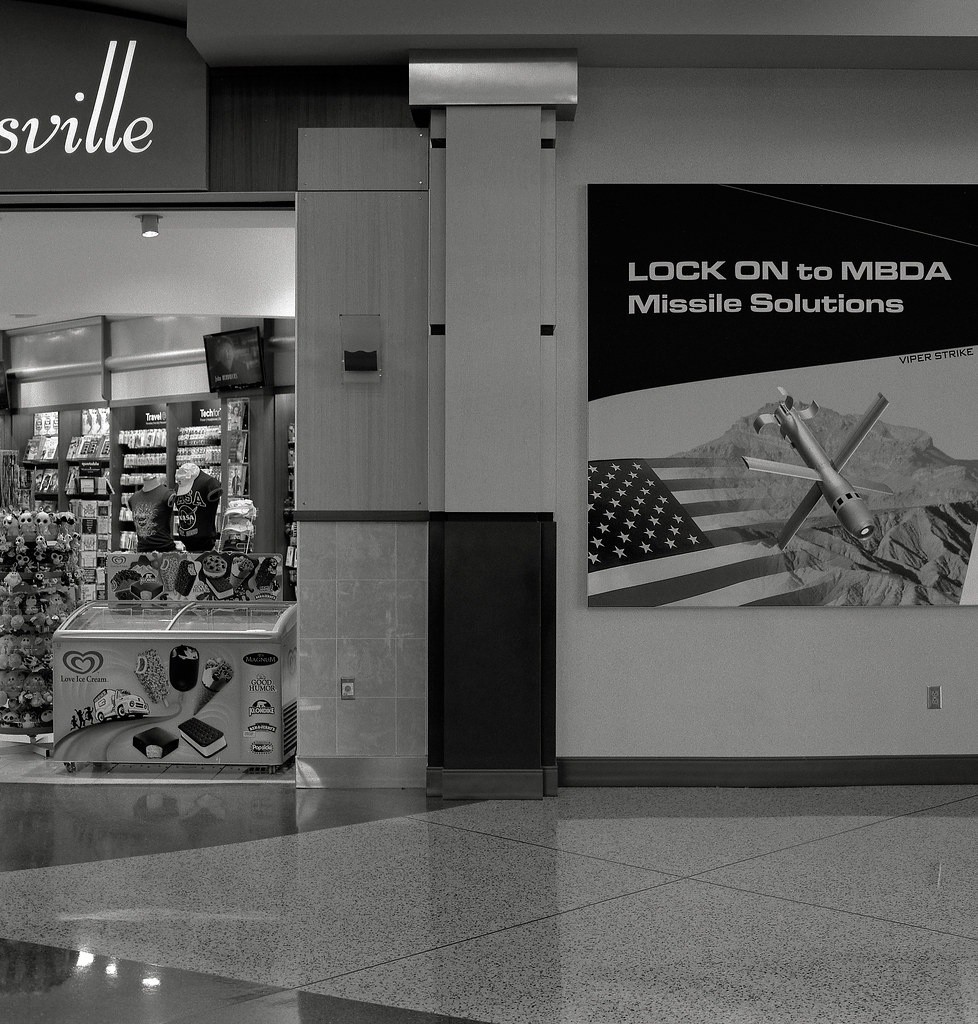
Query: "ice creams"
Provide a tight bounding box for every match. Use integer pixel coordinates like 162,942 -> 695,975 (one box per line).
193,657 -> 234,716
229,555 -> 254,590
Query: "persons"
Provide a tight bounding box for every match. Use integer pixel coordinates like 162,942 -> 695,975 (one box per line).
175,463 -> 222,553
128,477 -> 176,553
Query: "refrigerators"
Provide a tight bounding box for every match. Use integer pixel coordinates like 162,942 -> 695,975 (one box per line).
52,599 -> 298,774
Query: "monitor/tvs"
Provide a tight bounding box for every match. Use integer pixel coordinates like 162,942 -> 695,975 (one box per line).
203,326 -> 268,393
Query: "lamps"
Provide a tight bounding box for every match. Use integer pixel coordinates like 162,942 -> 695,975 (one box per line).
135,214 -> 164,238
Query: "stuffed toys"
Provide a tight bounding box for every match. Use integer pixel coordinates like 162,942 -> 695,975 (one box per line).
0,509 -> 84,729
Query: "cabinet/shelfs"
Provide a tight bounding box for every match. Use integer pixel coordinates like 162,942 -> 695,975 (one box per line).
22,397 -> 251,555
